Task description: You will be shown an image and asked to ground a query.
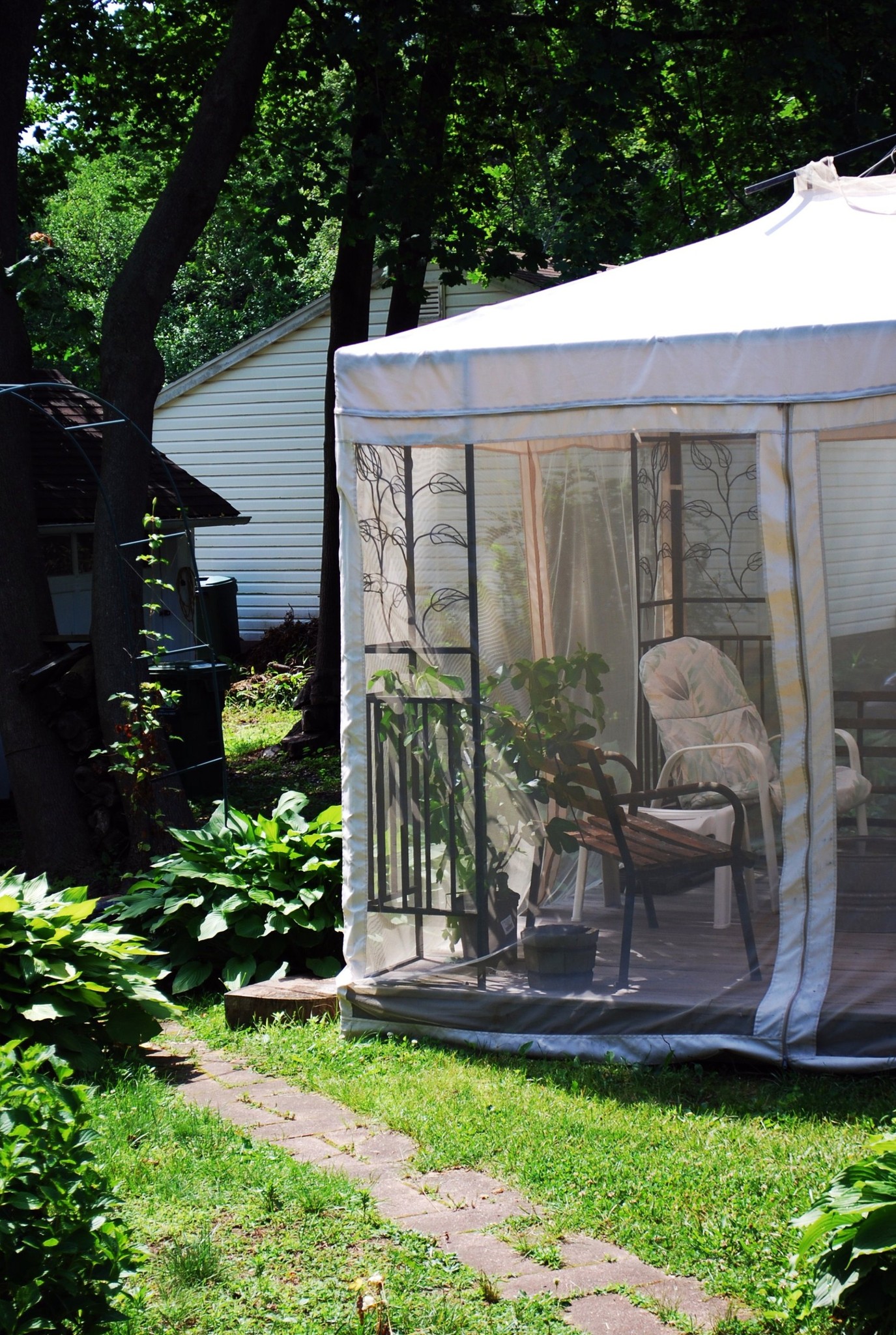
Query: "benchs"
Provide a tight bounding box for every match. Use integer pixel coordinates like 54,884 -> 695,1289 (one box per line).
497,718 -> 768,992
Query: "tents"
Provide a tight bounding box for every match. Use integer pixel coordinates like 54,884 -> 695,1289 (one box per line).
330,166 -> 896,1070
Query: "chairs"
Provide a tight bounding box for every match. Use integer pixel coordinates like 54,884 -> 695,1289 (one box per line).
641,630 -> 874,908
